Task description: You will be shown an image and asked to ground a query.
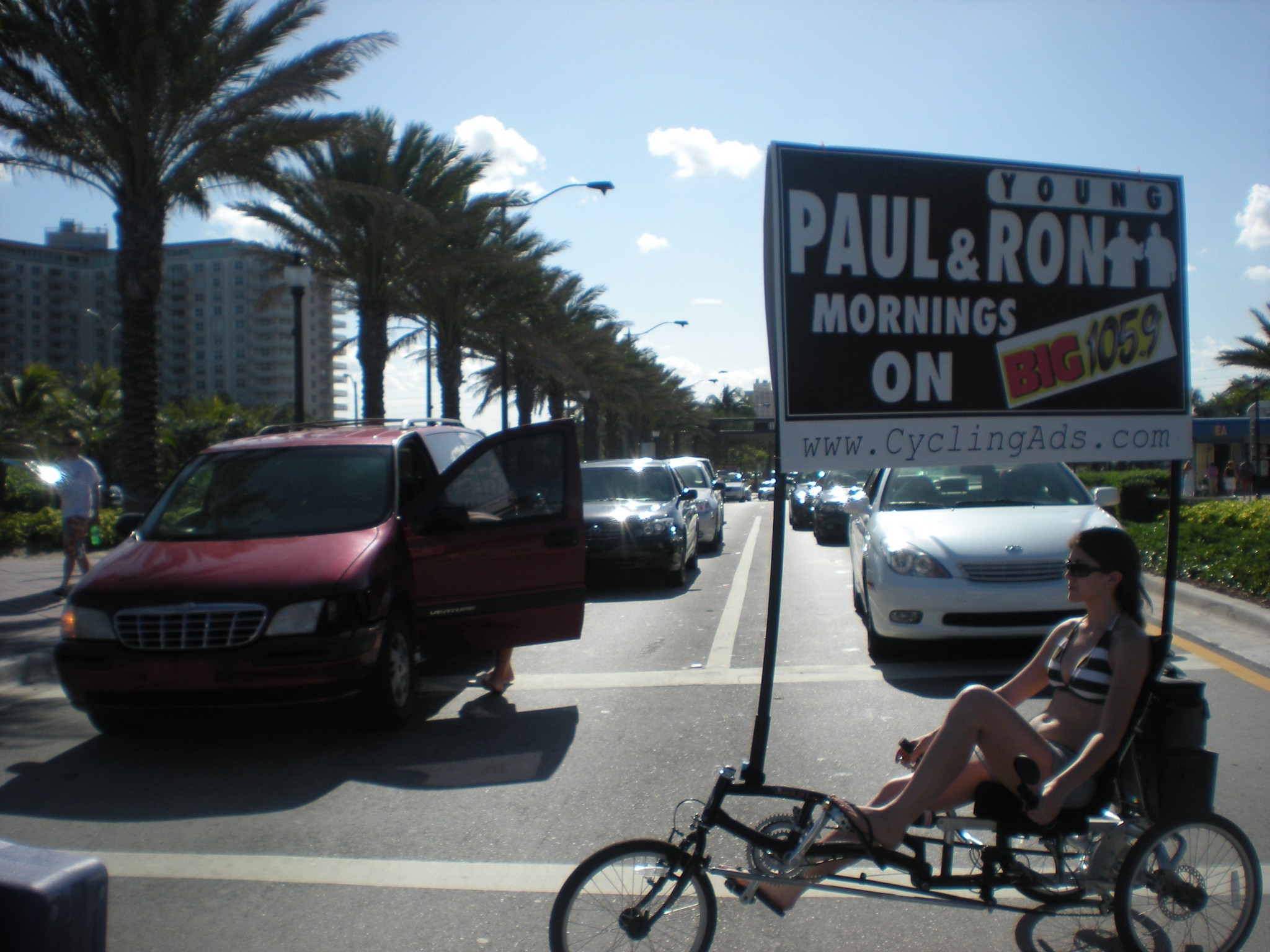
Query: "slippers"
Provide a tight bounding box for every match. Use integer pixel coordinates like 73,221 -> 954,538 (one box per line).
827,795 -> 891,871
723,867 -> 785,919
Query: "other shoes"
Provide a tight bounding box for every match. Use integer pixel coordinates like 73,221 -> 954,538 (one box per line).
53,586 -> 65,596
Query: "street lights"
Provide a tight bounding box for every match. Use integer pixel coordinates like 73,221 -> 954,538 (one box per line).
674,378 -> 719,458
86,307 -> 122,369
343,373 -> 358,426
623,319 -> 689,458
283,250 -> 316,434
499,179 -> 614,430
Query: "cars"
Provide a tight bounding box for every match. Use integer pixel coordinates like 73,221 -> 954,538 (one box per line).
0,439 -> 124,513
840,467 -> 1125,661
661,455 -> 927,553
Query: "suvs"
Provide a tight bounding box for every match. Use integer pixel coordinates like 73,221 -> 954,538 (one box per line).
50,410 -> 585,731
579,455 -> 699,589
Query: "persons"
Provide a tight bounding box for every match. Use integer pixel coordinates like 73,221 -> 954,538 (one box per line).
48,428 -> 102,596
1183,457 -> 1255,503
481,647 -> 514,695
716,526 -> 1150,918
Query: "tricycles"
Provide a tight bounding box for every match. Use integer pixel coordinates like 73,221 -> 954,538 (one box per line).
543,143 -> 1264,952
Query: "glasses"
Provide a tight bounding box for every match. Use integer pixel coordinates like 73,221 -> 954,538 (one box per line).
63,441 -> 76,447
1065,560 -> 1106,579
